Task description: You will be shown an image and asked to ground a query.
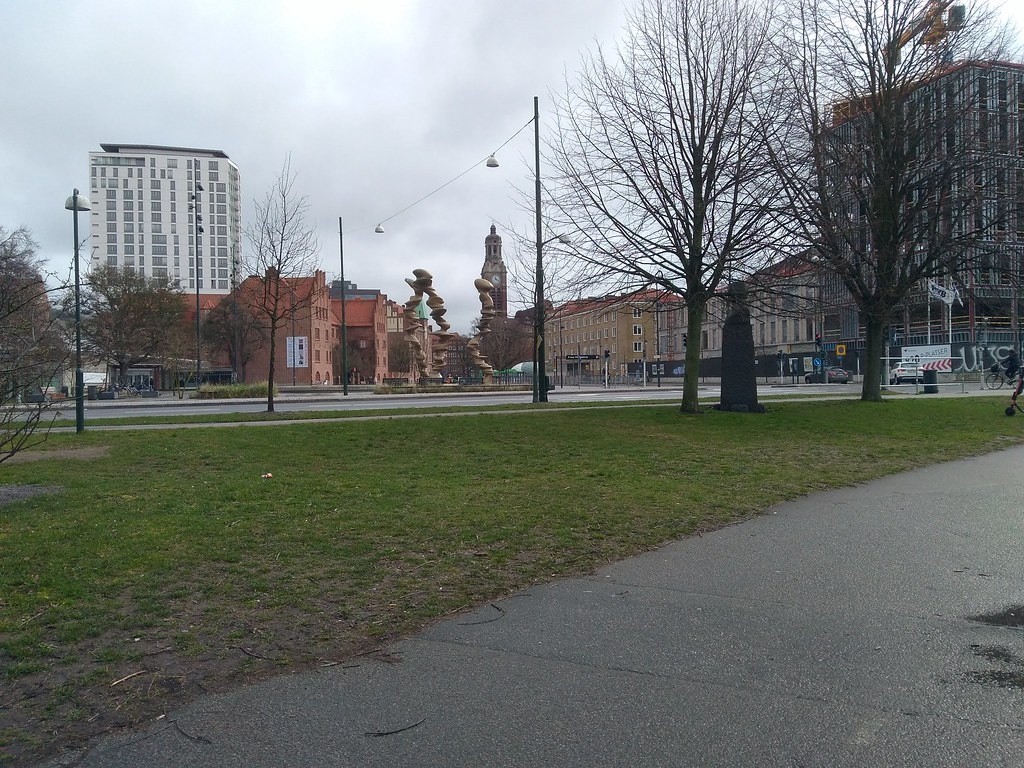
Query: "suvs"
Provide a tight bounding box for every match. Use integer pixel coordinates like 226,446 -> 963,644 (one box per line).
890,362 -> 924,385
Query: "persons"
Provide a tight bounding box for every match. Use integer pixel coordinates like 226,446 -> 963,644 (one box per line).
1001,349 -> 1019,379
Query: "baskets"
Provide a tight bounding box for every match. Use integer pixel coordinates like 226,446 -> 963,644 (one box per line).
991,363 -> 999,372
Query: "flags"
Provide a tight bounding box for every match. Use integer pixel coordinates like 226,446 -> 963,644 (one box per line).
929,280 -> 954,306
950,278 -> 963,306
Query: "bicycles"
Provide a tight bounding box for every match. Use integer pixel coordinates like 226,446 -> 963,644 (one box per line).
985,361 -> 1024,390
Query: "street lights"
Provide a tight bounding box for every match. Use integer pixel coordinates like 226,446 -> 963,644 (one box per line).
533,231 -> 571,405
65,188 -> 95,434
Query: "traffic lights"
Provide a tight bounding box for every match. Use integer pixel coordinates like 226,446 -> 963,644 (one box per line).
683,333 -> 687,347
817,333 -> 822,346
605,349 -> 611,358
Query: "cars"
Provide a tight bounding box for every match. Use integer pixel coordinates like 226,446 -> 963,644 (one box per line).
805,366 -> 848,384
420,371 -> 443,384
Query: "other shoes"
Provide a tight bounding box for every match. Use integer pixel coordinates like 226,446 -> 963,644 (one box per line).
1005,378 -> 1014,383
